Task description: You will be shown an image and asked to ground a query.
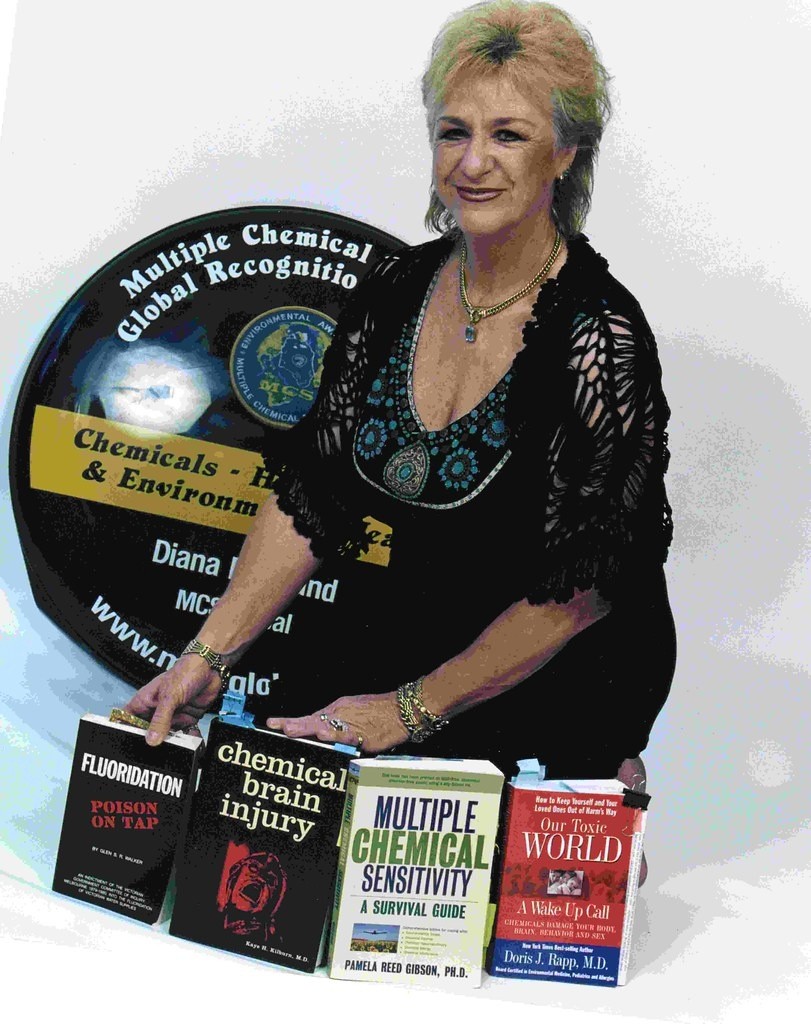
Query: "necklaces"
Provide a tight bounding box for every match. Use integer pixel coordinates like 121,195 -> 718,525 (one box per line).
458,227 -> 565,342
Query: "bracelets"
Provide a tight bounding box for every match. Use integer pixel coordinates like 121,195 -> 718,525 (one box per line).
180,638 -> 232,690
396,677 -> 451,743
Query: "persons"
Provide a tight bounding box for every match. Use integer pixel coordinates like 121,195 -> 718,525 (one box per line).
123,0 -> 677,890
548,870 -> 582,896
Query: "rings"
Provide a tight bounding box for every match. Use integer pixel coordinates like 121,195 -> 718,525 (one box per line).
356,732 -> 364,748
330,719 -> 345,732
320,713 -> 328,723
183,724 -> 199,734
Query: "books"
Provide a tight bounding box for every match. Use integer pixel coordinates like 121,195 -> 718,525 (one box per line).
51,716 -> 648,989
